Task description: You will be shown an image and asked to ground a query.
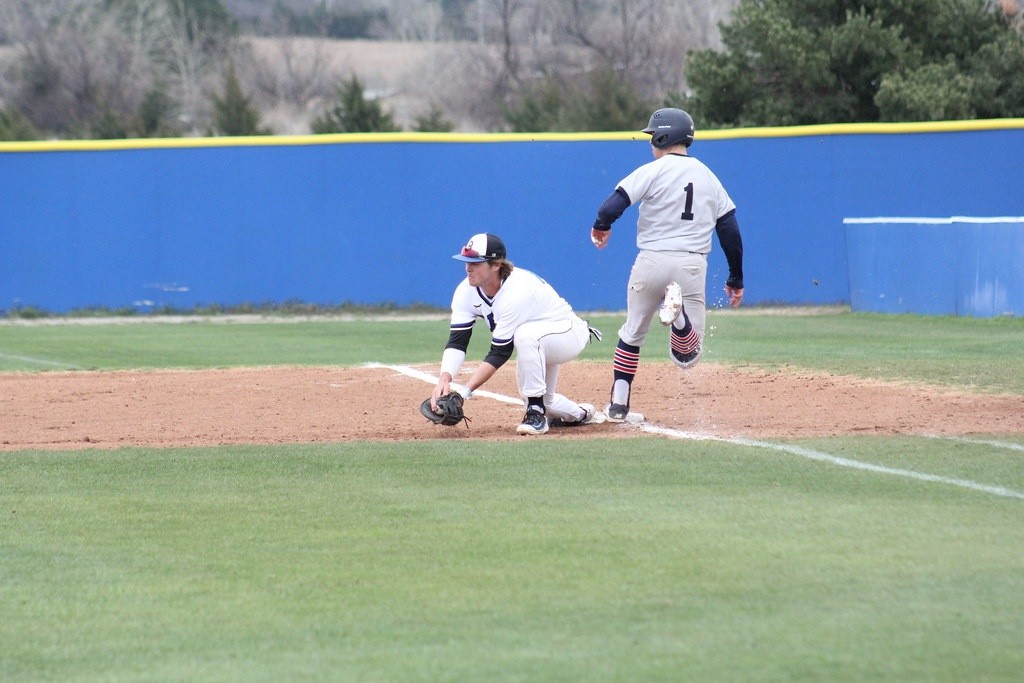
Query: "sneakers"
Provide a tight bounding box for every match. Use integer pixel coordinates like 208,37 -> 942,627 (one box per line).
551,403 -> 595,426
602,403 -> 630,422
659,282 -> 683,326
517,405 -> 549,434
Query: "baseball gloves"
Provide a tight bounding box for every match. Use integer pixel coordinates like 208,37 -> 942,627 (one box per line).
419,391 -> 464,426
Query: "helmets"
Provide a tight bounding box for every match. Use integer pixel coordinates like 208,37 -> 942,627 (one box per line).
642,108 -> 694,149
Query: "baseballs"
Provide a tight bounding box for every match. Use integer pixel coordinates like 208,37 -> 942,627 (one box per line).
434,405 -> 444,414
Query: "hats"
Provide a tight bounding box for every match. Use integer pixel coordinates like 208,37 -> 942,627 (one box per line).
452,232 -> 506,263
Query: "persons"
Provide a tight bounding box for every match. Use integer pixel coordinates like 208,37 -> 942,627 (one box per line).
430,232 -> 602,435
591,108 -> 745,419
